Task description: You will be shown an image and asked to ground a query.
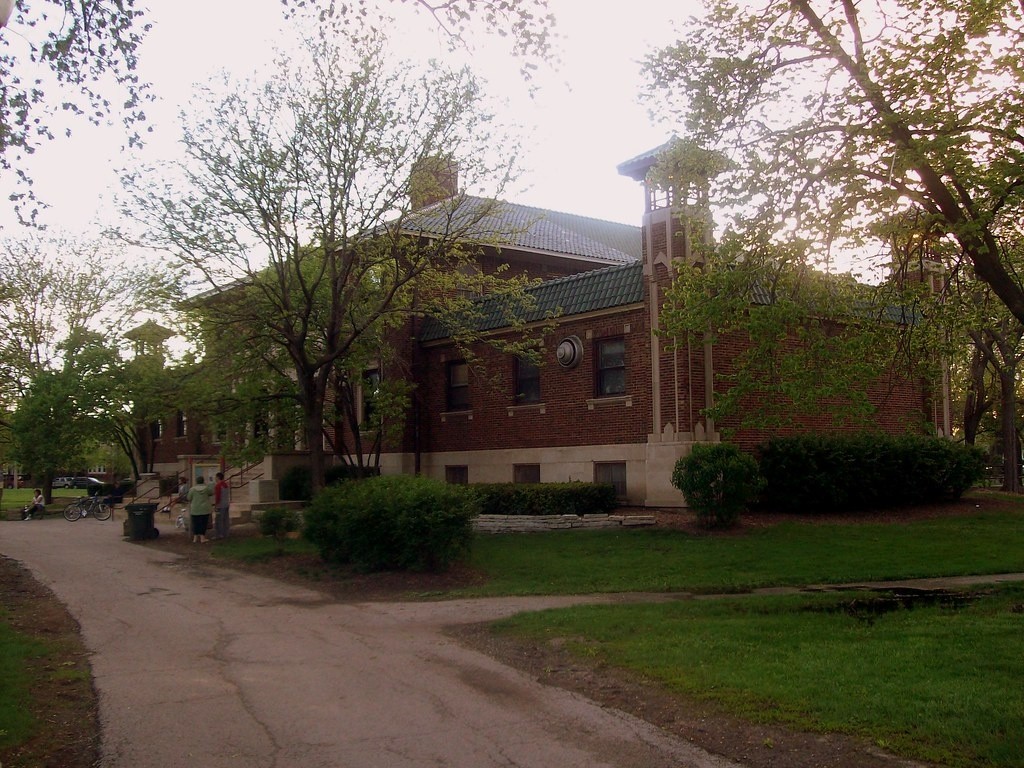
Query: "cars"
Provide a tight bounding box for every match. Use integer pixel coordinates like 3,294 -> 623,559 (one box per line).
4,475 -> 24,489
52,476 -> 74,489
71,477 -> 104,489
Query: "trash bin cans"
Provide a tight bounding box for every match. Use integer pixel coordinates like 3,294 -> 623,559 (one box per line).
125,501 -> 159,540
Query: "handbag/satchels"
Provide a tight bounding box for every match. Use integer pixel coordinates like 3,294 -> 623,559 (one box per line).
177,495 -> 187,503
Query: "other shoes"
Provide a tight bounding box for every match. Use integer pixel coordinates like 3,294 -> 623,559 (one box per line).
201,539 -> 210,543
24,509 -> 30,512
211,534 -> 224,539
225,537 -> 231,541
193,540 -> 197,542
24,517 -> 31,520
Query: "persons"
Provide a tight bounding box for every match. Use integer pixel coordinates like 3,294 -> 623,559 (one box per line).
99,480 -> 124,509
21,489 -> 45,520
212,473 -> 230,540
187,476 -> 212,543
160,477 -> 189,513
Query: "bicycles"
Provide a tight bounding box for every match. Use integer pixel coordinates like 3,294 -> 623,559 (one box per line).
63,490 -> 111,522
176,507 -> 189,532
